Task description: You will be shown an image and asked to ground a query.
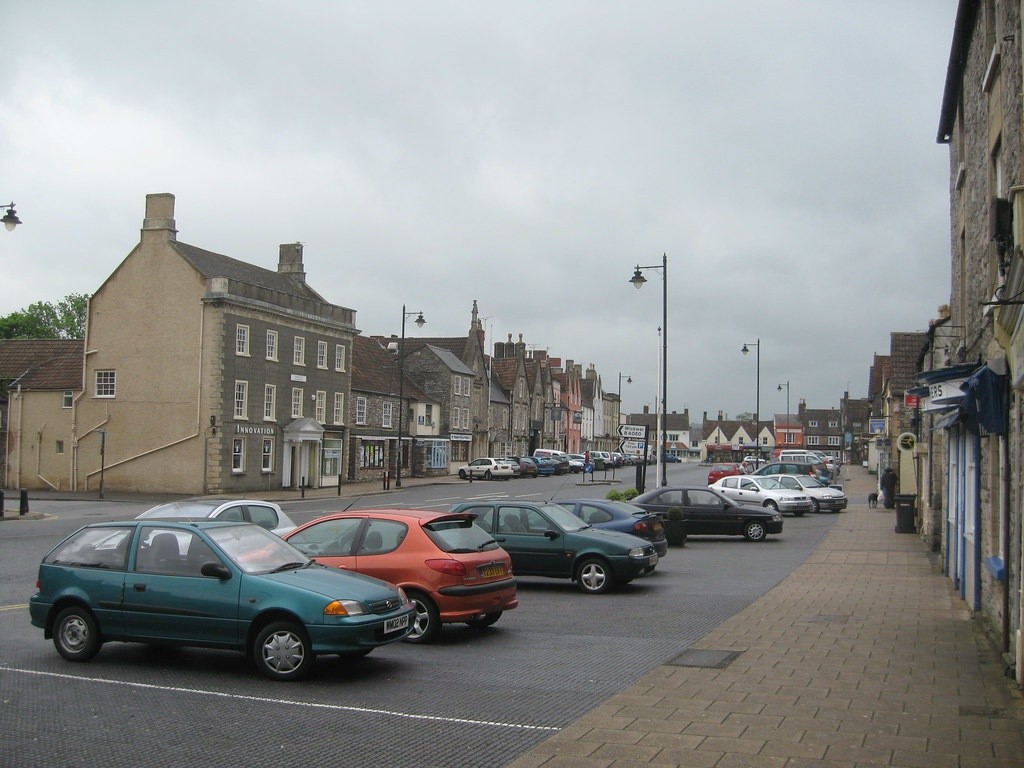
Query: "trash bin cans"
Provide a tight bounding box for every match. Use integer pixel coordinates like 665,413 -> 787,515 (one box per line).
894,492 -> 917,534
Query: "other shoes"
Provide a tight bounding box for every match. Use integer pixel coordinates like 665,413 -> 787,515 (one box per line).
890,505 -> 894,508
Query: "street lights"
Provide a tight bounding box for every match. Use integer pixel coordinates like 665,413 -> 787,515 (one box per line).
617,372 -> 633,452
776,380 -> 790,450
629,252 -> 668,487
394,304 -> 427,488
740,337 -> 761,470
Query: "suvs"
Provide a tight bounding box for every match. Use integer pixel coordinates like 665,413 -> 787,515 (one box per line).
661,453 -> 681,463
744,456 -> 766,464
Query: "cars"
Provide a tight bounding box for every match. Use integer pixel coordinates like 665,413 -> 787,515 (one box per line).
626,484 -> 785,542
93,496 -> 306,559
235,507 -> 519,645
766,472 -> 848,514
707,475 -> 813,517
29,516 -> 418,683
707,463 -> 743,485
448,500 -> 659,594
458,457 -> 515,481
527,498 -> 668,559
749,449 -> 841,486
501,448 -> 657,481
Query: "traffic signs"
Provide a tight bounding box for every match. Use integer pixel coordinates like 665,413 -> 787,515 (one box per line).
618,439 -> 645,456
615,423 -> 646,440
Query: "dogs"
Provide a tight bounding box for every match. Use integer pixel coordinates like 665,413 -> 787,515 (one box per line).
868,493 -> 878,509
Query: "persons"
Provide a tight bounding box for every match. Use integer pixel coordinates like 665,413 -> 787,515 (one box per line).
585,451 -> 589,461
880,467 -> 898,509
739,462 -> 747,473
